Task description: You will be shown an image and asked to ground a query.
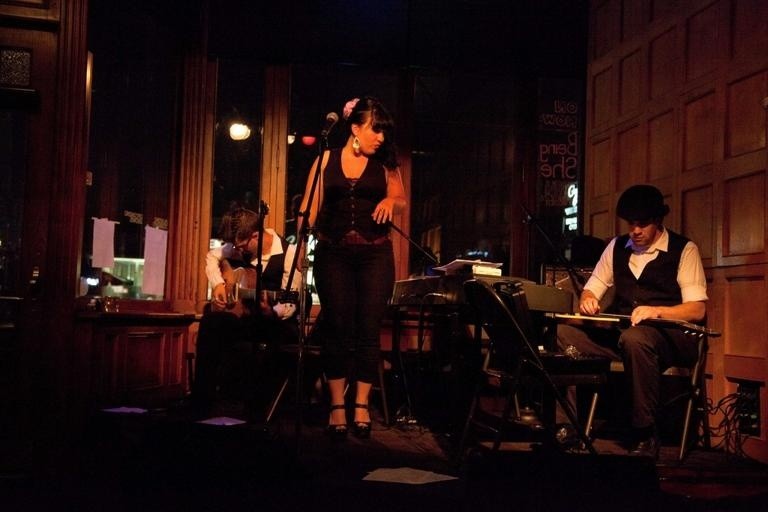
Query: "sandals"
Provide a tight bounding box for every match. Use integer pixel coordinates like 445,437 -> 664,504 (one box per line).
327,401 -> 349,437
352,403 -> 372,435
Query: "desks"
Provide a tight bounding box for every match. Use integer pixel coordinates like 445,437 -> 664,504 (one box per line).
78,311 -> 197,408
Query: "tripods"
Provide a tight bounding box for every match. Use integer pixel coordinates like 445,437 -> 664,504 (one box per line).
263,162 -> 326,426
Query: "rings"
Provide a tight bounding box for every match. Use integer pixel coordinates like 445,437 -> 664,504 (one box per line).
380,208 -> 385,212
216,298 -> 218,301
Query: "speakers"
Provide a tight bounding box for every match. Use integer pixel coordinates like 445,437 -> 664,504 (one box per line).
113,222 -> 145,258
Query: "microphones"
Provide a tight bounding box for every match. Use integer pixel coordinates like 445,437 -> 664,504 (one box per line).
520,205 -> 535,222
259,200 -> 271,215
322,111 -> 339,137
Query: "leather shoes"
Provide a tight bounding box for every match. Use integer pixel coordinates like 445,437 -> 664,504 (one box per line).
530,426 -> 585,452
630,427 -> 662,458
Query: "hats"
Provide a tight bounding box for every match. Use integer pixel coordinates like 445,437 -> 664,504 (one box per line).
614,185 -> 670,221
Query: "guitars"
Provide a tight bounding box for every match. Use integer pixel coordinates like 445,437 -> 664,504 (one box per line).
211,258 -> 301,316
546,309 -> 721,339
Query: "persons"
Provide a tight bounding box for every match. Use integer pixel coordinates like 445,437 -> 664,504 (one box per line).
527,185 -> 710,458
293,95 -> 408,440
167,208 -> 305,427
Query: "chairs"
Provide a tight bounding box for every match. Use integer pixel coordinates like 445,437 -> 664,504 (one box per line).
245,287 -> 314,428
579,299 -> 722,462
454,276 -> 598,467
263,311 -> 413,431
